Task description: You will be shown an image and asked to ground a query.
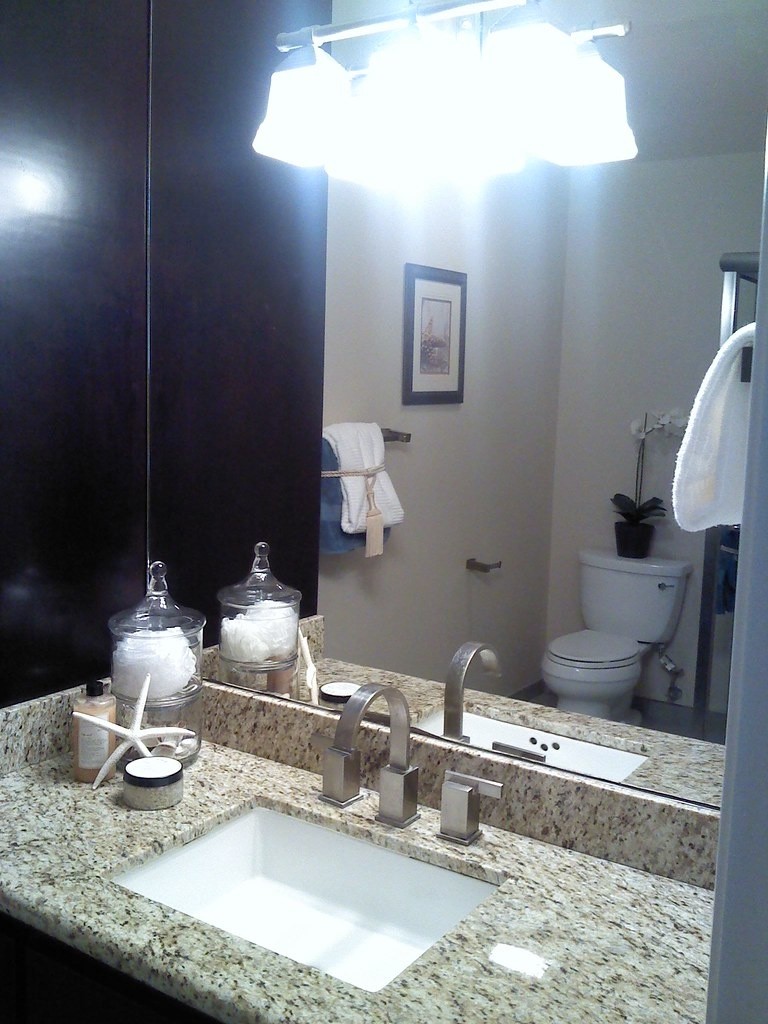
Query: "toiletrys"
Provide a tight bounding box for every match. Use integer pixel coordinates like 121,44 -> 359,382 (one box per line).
321,682 -> 362,710
70,677 -> 118,785
124,753 -> 184,809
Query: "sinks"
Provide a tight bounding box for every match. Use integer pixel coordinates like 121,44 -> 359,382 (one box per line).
99,790 -> 509,991
415,696 -> 647,780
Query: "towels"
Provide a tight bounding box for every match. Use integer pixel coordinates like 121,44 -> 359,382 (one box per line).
670,323 -> 756,533
713,526 -> 737,613
319,421 -> 403,556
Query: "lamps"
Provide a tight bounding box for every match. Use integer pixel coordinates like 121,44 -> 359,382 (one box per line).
254,0 -> 527,165
533,24 -> 638,166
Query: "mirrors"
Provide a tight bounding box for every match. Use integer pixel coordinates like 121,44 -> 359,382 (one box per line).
148,0 -> 768,819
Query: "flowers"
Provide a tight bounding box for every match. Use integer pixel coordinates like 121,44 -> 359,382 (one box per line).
609,408 -> 687,521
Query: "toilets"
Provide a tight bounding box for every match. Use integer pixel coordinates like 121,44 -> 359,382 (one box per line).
539,549 -> 693,727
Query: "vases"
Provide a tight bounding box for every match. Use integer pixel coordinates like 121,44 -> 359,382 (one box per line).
615,522 -> 655,559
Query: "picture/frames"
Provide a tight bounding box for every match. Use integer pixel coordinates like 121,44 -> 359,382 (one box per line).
402,263 -> 468,405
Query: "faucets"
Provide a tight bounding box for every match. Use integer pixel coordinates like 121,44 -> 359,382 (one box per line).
320,684 -> 422,831
439,638 -> 503,745
467,557 -> 504,575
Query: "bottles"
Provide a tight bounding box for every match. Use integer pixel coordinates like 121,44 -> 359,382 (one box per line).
71,681 -> 116,781
107,561 -> 207,768
216,542 -> 303,698
